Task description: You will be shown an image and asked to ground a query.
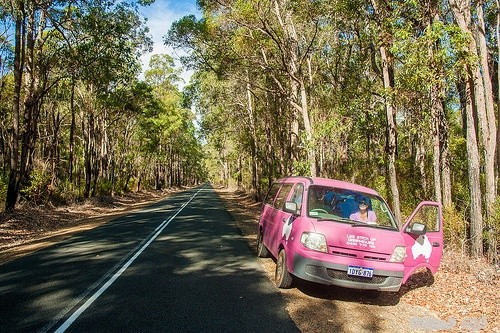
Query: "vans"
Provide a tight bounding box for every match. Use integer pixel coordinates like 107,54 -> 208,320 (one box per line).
255,176 -> 444,296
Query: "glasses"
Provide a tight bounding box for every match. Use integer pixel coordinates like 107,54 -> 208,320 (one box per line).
359,202 -> 367,205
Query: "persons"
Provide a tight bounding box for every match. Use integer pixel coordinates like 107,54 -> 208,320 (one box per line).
349,197 -> 379,226
311,191 -> 344,218
